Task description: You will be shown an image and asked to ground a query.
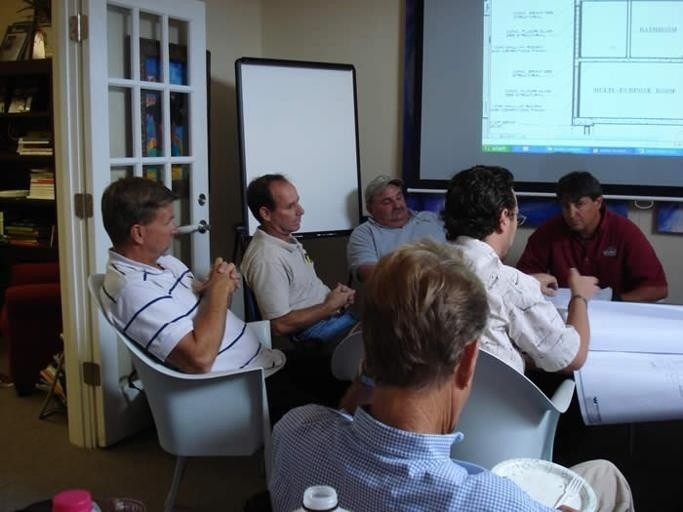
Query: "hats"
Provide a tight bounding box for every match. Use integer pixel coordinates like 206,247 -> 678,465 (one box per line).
365,175 -> 404,203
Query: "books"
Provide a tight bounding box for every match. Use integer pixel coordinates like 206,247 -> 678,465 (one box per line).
1,135 -> 56,246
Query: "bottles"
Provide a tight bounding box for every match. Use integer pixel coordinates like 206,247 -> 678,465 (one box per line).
293,486 -> 350,512
53,489 -> 102,512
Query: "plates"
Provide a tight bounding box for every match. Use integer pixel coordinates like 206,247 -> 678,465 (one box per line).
491,457 -> 597,512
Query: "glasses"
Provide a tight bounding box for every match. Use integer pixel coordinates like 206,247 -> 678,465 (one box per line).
508,211 -> 526,226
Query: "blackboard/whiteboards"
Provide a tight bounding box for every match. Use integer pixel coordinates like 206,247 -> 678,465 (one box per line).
235,56 -> 363,240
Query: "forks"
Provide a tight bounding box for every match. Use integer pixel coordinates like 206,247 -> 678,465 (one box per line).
553,476 -> 584,510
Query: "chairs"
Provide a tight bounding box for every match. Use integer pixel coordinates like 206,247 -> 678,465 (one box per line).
1,260 -> 60,400
331,312 -> 575,474
86,271 -> 276,508
244,263 -> 336,406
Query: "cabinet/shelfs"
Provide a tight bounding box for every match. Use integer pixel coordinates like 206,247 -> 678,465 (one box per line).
1,65 -> 55,243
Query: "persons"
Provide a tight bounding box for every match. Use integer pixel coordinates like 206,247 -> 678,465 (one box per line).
435,164 -> 600,376
239,175 -> 364,375
101,176 -> 351,416
347,175 -> 449,293
266,236 -> 634,511
515,171 -> 669,303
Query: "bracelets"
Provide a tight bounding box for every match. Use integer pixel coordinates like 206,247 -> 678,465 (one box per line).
565,295 -> 590,304
